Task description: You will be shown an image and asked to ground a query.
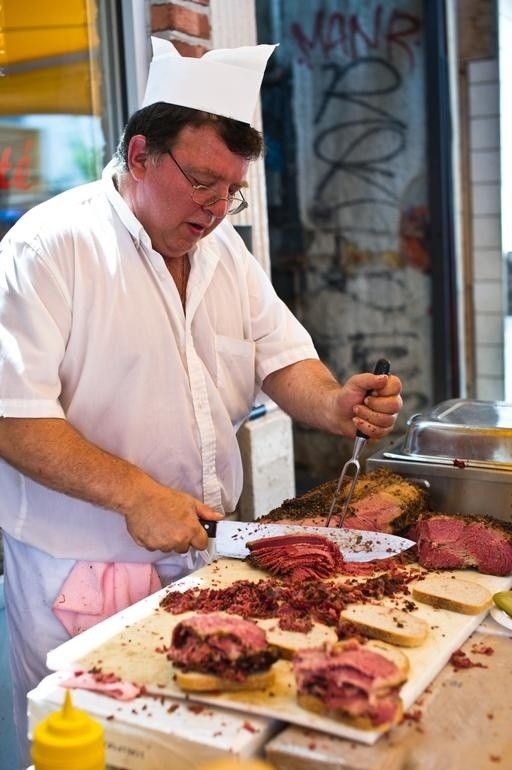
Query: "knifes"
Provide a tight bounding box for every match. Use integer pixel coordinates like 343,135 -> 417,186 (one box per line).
199,518 -> 417,565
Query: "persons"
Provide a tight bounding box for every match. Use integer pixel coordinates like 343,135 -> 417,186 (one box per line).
0,36 -> 403,770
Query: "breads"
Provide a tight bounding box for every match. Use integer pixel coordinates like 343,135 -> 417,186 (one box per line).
330,638 -> 410,688
340,602 -> 429,648
294,690 -> 404,733
412,577 -> 494,615
247,615 -> 338,660
175,669 -> 276,692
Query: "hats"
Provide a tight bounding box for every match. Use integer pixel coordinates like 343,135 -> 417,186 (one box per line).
140,36 -> 279,123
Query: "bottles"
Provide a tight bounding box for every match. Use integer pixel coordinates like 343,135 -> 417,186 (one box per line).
25,690 -> 106,770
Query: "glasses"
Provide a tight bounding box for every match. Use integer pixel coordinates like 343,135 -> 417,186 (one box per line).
169,151 -> 247,215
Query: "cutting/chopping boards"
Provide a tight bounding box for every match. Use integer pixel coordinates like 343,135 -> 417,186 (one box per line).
44,556 -> 511,747
